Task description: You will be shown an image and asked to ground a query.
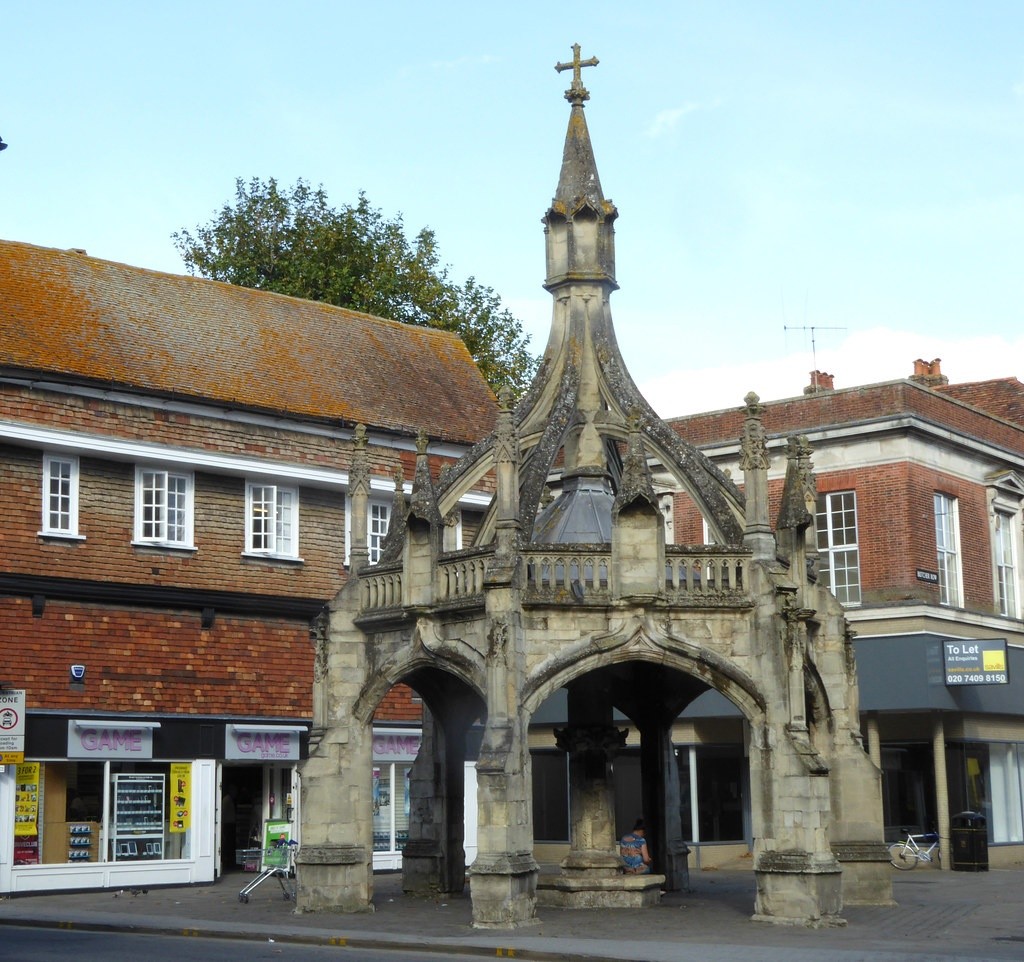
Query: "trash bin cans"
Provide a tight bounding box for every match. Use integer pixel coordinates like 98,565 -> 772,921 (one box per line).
950,811 -> 989,873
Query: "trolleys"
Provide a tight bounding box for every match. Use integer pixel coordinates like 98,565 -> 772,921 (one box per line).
238,840 -> 297,903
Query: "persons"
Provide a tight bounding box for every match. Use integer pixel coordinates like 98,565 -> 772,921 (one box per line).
620,819 -> 666,895
275,834 -> 288,848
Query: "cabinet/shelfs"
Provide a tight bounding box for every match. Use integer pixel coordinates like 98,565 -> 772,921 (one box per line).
110,773 -> 166,861
69,831 -> 93,859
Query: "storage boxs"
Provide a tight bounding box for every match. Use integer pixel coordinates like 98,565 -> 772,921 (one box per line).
235,848 -> 261,866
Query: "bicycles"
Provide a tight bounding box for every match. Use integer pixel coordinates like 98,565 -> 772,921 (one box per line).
889,824 -> 954,871
